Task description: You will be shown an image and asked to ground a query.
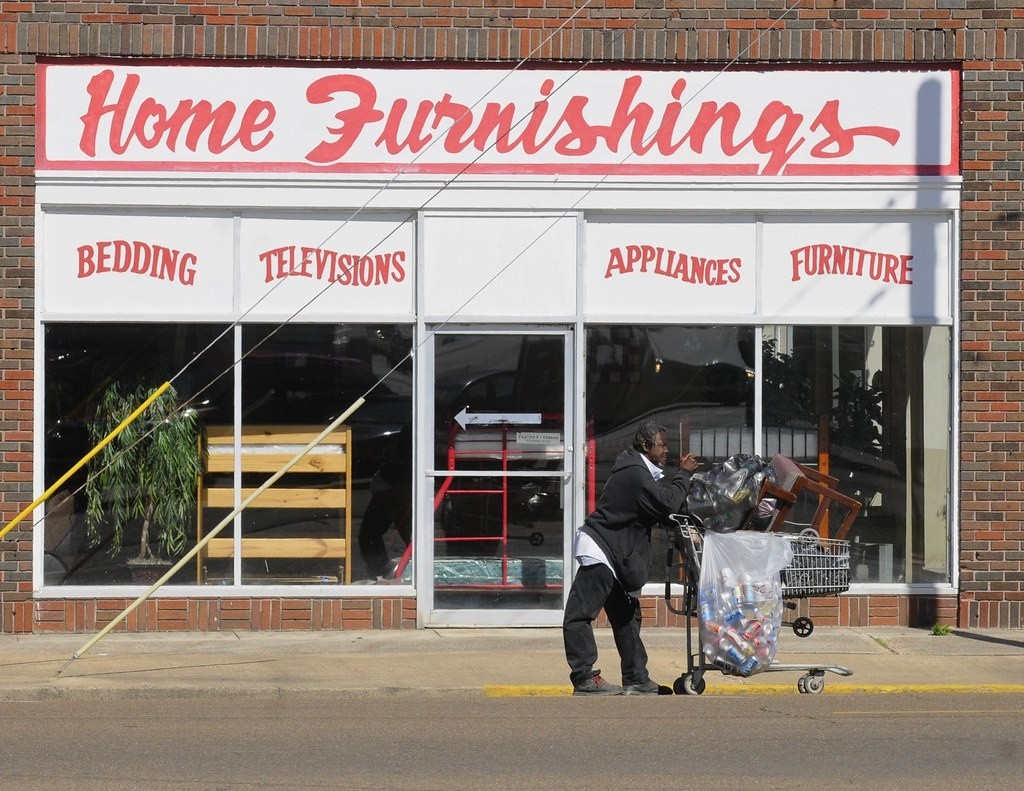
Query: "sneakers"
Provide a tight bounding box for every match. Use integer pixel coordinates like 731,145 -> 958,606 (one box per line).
622,677 -> 674,696
572,669 -> 624,696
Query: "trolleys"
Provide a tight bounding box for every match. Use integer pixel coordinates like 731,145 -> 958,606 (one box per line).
670,513 -> 857,697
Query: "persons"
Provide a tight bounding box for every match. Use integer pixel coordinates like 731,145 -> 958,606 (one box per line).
563,423 -> 698,697
356,425 -> 411,580
